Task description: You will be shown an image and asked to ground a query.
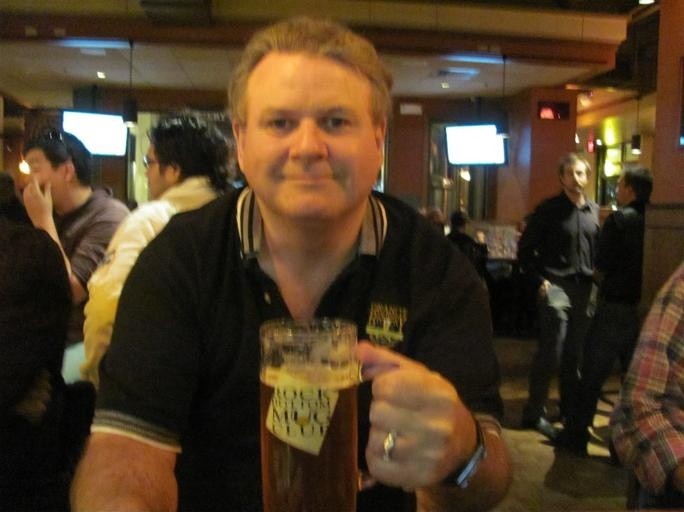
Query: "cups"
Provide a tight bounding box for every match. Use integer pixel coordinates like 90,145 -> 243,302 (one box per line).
253,316 -> 404,512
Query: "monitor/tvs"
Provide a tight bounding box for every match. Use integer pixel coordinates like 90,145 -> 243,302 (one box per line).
444,121 -> 509,168
59,108 -> 130,158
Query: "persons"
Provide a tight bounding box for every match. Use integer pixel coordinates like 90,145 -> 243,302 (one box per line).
605,260 -> 683,511
517,156 -> 602,435
21,125 -> 129,339
445,210 -> 477,246
538,166 -> 654,450
0,170 -> 99,512
69,15 -> 514,510
81,113 -> 236,391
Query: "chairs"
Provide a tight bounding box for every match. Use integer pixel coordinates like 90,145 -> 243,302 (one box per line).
468,241 -> 489,276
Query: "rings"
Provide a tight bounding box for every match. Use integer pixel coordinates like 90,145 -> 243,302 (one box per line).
383,428 -> 395,459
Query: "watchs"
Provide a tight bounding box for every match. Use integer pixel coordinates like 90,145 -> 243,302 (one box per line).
440,415 -> 490,488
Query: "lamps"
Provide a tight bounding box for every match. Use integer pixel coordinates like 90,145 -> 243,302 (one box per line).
631,94 -> 643,156
120,40 -> 139,126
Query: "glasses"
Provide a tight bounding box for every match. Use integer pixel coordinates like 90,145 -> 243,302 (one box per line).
144,156 -> 166,167
